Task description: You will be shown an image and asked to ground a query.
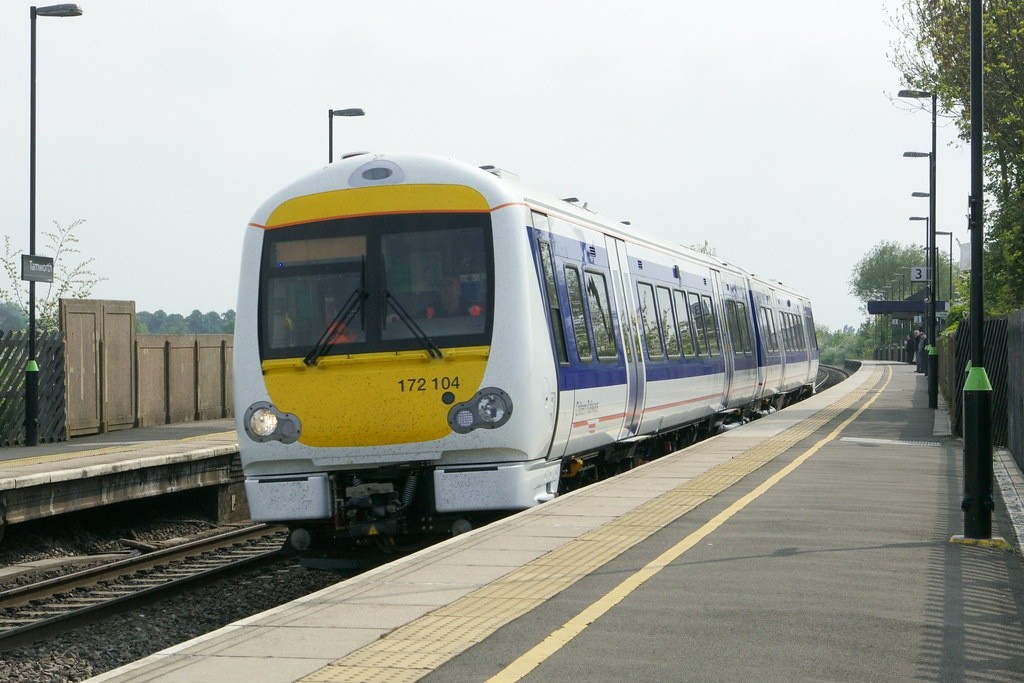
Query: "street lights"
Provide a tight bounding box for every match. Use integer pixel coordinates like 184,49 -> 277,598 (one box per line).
873,151 -> 952,376
327,107 -> 367,164
20,2 -> 84,446
897,88 -> 940,410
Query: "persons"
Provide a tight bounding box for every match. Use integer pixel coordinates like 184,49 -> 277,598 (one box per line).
913,330 -> 922,371
917,332 -> 928,373
906,334 -> 915,365
415,260 -> 477,322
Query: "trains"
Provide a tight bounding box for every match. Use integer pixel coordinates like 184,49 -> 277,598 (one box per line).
230,148 -> 824,575
